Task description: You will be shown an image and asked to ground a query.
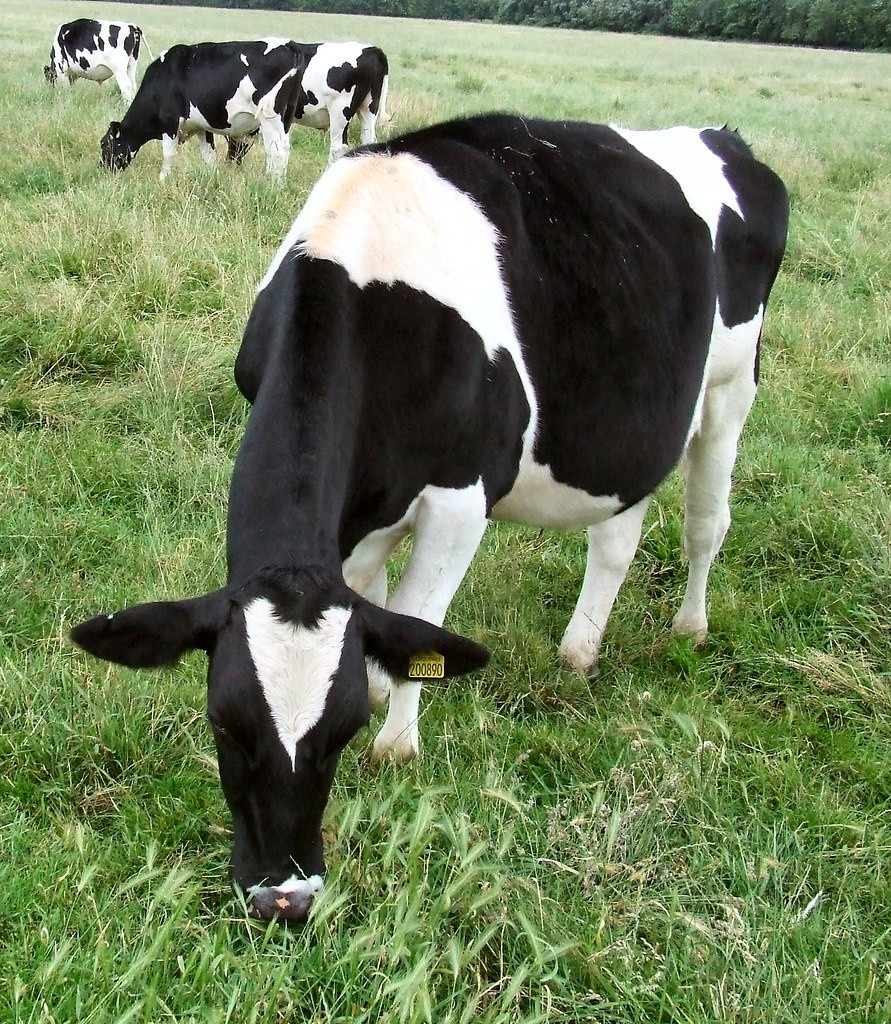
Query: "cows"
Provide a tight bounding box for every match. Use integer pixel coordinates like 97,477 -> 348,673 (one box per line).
69,106 -> 793,922
42,18 -> 154,111
99,38 -> 391,186
